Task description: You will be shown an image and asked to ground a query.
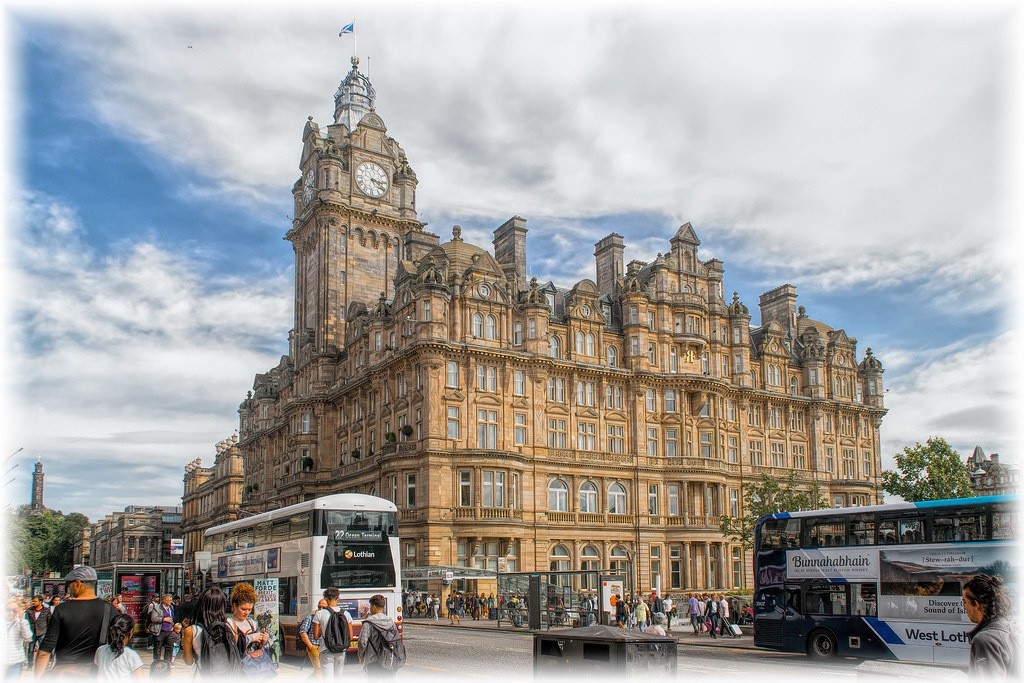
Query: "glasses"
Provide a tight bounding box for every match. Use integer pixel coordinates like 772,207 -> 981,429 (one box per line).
318,605 -> 326,609
32,600 -> 40,602
6,608 -> 18,613
66,579 -> 84,585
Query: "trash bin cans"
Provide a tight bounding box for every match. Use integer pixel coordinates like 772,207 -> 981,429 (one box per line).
533,625 -> 680,676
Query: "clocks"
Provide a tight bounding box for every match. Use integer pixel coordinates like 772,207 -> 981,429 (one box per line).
302,167 -> 316,207
355,162 -> 391,199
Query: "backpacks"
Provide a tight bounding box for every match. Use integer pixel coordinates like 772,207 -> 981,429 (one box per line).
623,602 -> 631,617
139,601 -> 159,628
296,613 -> 314,651
191,621 -> 242,682
362,620 -> 406,671
322,606 -> 351,653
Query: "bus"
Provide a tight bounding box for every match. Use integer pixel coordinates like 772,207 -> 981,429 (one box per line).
202,493 -> 403,661
752,493 -> 1021,668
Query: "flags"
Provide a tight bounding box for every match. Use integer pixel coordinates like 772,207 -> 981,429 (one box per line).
339,23 -> 353,37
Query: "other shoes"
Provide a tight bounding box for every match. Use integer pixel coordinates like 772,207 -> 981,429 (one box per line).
171,663 -> 176,668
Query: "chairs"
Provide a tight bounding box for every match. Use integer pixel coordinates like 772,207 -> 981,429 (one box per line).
778,531 -> 984,549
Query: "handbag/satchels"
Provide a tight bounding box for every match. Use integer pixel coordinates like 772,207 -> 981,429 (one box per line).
241,648 -> 278,681
449,602 -> 454,609
419,602 -> 426,612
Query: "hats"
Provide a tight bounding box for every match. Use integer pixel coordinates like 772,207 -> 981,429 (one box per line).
651,592 -> 657,595
65,566 -> 97,581
154,593 -> 159,598
653,611 -> 665,623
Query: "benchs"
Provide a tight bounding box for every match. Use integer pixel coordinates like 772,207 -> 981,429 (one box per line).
550,616 -> 584,628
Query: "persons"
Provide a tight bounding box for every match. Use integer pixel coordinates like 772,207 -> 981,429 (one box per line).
6,566 -> 399,680
962,574 -> 1016,676
615,590 -> 676,637
578,589 -> 597,626
686,592 -> 754,639
445,590 -> 571,628
402,588 -> 440,622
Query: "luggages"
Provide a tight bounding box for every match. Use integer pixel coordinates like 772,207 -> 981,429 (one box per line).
704,617 -> 721,634
723,616 -> 743,638
